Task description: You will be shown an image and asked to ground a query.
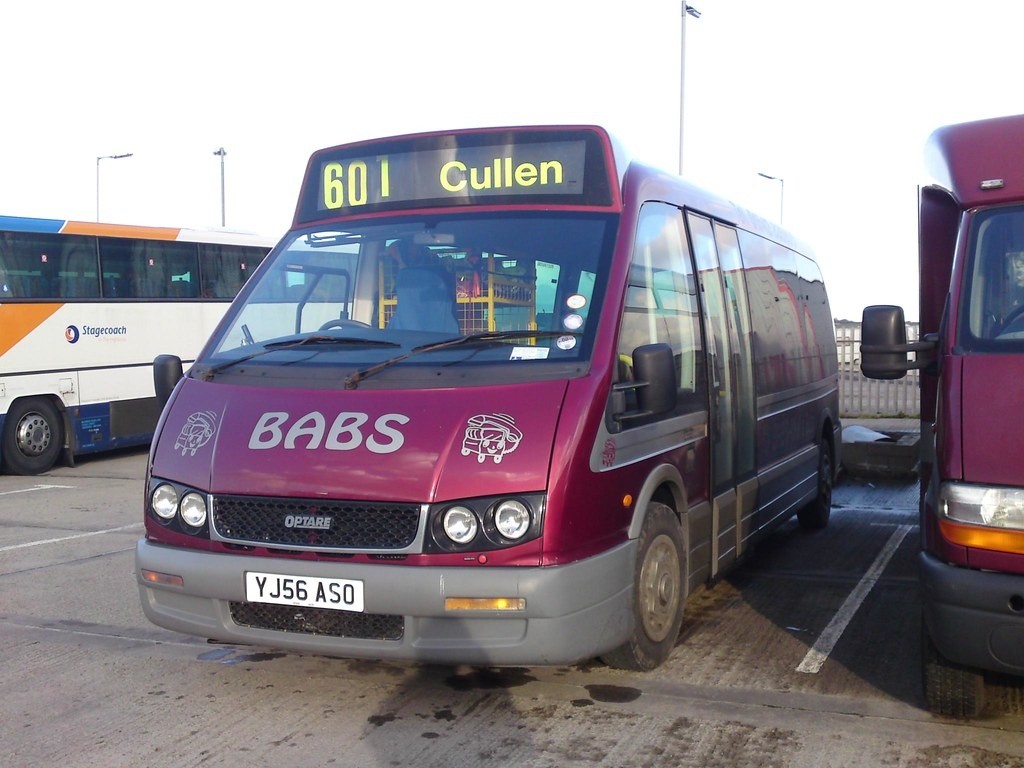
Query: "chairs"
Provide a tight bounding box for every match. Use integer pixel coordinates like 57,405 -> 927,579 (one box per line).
13,275 -> 190,299
384,267 -> 459,334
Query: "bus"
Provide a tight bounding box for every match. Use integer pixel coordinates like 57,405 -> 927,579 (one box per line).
0,212 -> 362,478
859,116 -> 1023,720
137,121 -> 845,674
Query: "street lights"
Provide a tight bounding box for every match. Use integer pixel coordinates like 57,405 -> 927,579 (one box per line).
759,171 -> 784,227
213,147 -> 226,229
95,153 -> 134,224
678,0 -> 702,174
